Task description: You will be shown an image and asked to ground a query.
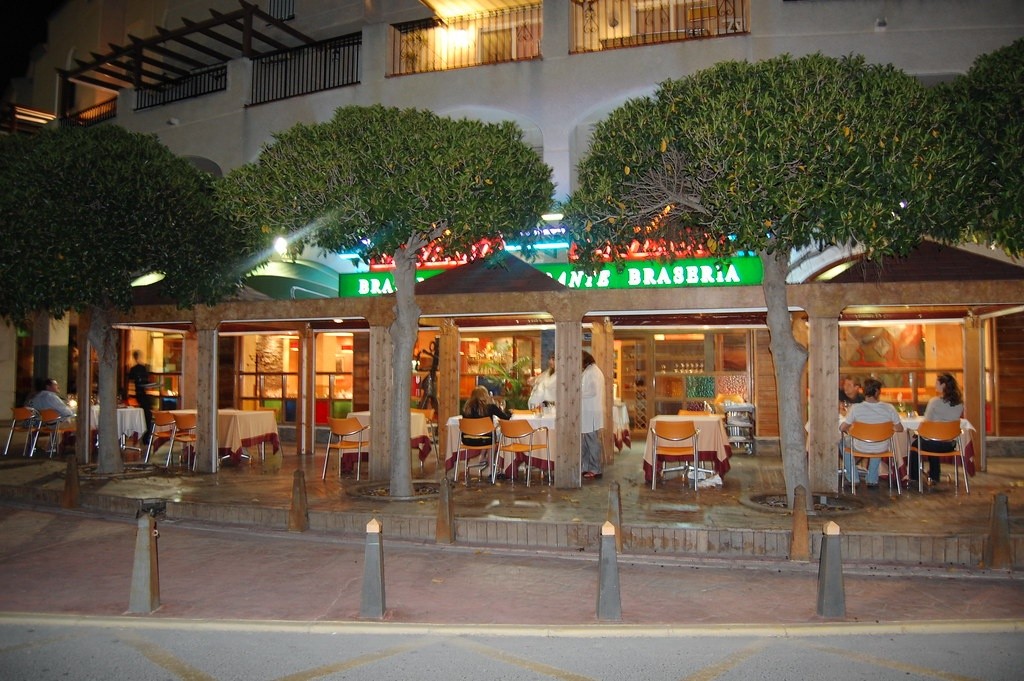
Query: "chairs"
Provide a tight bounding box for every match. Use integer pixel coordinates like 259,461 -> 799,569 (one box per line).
145,407 -> 284,472
322,416 -> 368,482
411,407 -> 439,473
841,422 -> 902,495
906,420 -> 970,495
453,408 -> 552,488
651,410 -> 714,492
3,406 -> 63,459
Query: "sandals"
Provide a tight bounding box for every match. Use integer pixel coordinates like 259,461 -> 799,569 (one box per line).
583,471 -> 602,478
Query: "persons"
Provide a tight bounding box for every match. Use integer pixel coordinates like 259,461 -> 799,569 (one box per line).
910,374 -> 963,486
34,377 -> 73,454
839,375 -> 865,403
128,350 -> 151,446
461,386 -> 513,478
528,353 -> 556,410
839,379 -> 904,487
581,350 -> 604,478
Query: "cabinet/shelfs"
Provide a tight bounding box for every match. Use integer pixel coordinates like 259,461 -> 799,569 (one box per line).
721,403 -> 755,454
461,358 -> 512,377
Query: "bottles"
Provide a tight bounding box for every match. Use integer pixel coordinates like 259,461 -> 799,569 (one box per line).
490,391 -> 494,403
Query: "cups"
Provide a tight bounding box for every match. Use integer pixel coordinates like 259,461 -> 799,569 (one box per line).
495,399 -> 506,411
843,403 -> 851,411
898,402 -> 906,412
530,403 -> 536,416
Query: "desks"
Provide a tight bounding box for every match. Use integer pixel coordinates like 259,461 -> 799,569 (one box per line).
70,406 -> 146,452
340,410 -> 431,473
644,414 -> 733,487
444,413 -> 555,480
613,403 -> 632,453
153,409 -> 279,468
804,413 -> 977,483
